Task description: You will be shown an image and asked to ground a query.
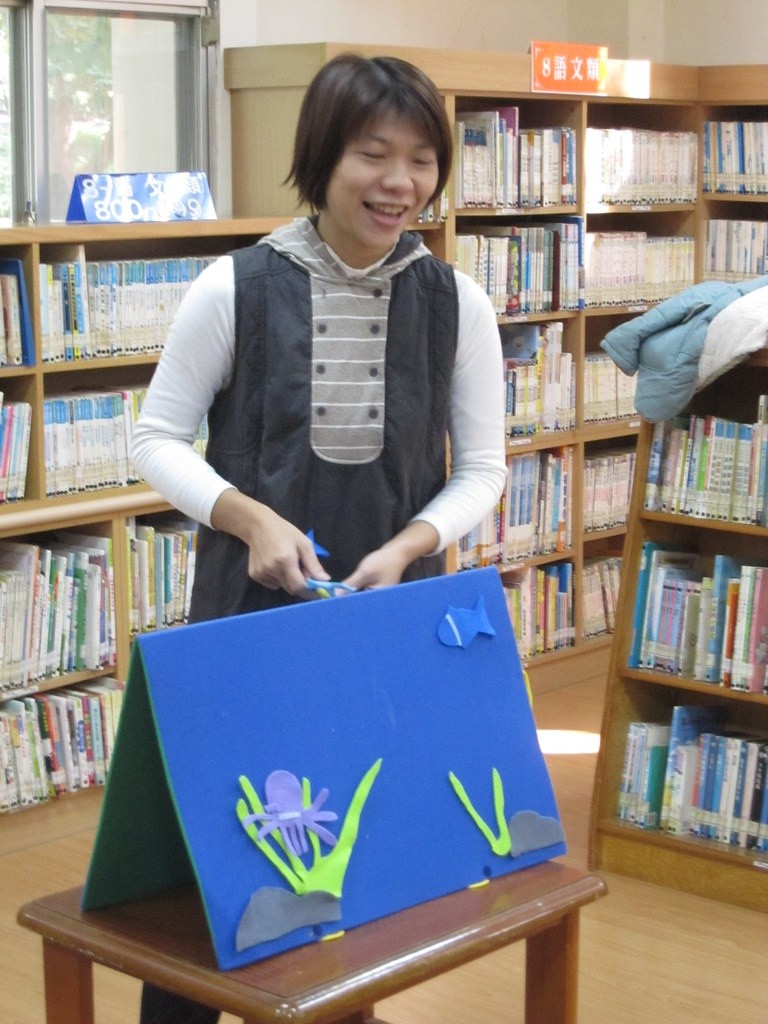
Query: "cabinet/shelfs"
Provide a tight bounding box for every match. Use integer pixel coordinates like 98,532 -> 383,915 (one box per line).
586,349 -> 767,911
0,45 -> 766,815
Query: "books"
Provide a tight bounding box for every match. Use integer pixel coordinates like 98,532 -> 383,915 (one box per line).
1,255 -> 208,811
419,108 -> 768,859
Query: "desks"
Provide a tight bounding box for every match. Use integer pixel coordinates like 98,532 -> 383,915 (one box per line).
16,854 -> 607,1024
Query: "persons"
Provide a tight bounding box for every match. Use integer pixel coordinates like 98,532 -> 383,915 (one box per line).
125,52 -> 508,1024
